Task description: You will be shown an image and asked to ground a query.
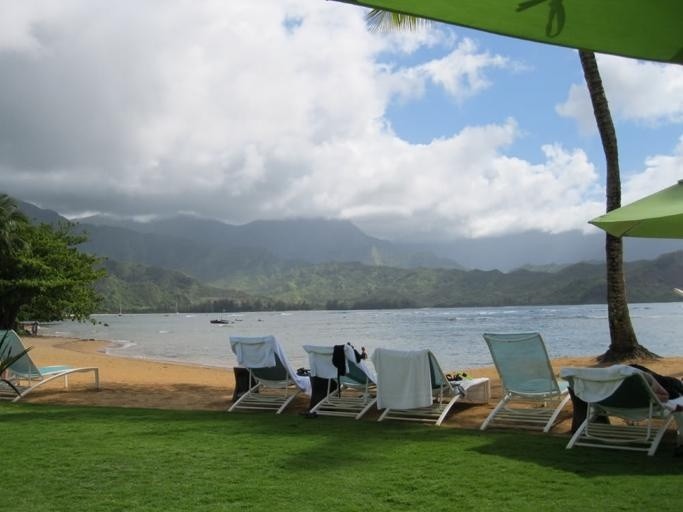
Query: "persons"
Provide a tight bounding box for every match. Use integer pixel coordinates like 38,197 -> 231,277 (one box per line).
628,363 -> 682,401
359,346 -> 368,360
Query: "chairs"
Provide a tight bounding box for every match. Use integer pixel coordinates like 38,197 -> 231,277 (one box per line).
0,328 -> 99,402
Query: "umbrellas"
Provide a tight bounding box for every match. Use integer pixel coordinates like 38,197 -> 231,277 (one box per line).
330,0 -> 682,65
588,179 -> 682,243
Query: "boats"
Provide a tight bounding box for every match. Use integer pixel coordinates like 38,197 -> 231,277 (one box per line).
210,319 -> 234,323
